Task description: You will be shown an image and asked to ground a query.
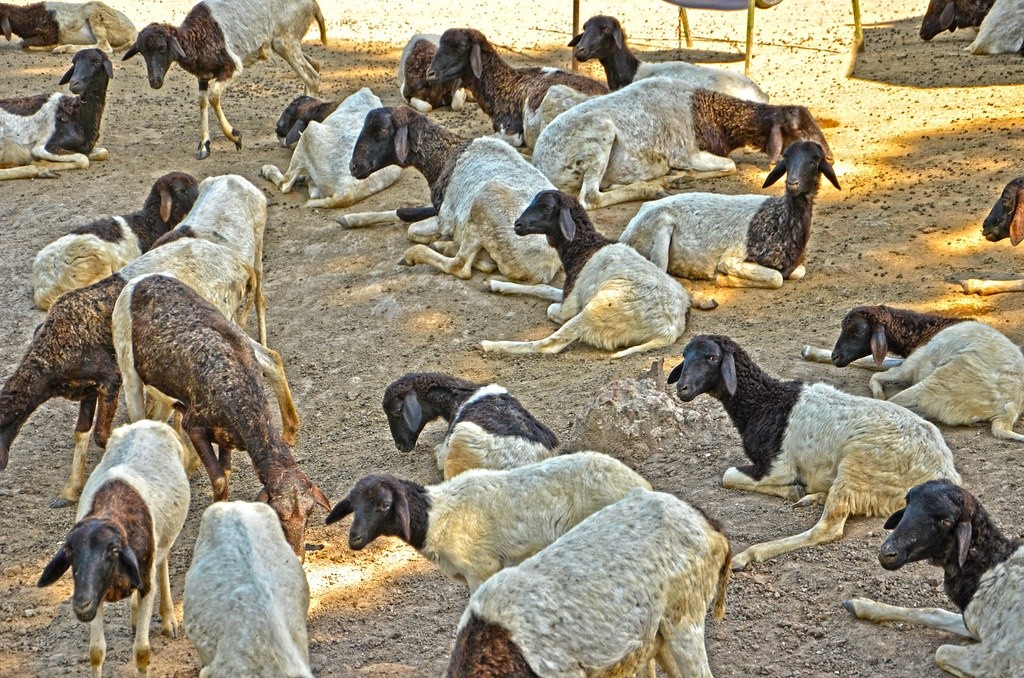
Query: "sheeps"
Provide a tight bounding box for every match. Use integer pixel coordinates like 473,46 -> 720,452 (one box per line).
182,500 -> 313,678
382,371 -> 562,481
444,487 -> 732,678
0,0 -> 443,341
666,335 -> 963,573
800,305 -> 1024,441
332,105 -> 563,285
0,236 -> 257,507
959,176 -> 1024,296
324,450 -> 653,595
842,478 -> 1024,678
619,140 -> 841,289
111,273 -> 332,566
37,420 -> 191,678
478,189 -> 693,359
